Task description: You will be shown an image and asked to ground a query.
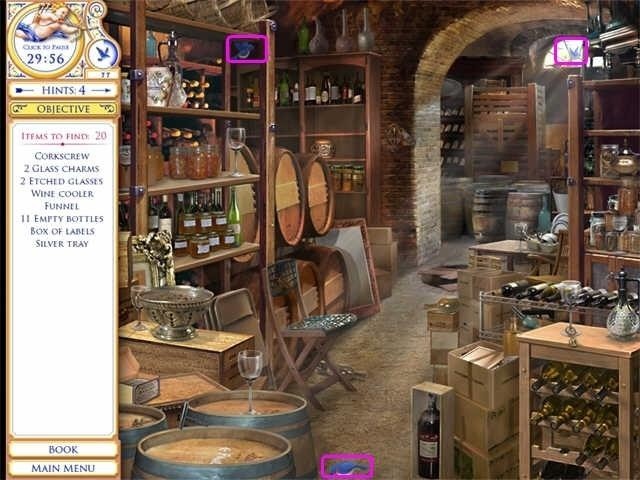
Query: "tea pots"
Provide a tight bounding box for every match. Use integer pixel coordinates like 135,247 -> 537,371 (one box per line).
158,30 -> 183,78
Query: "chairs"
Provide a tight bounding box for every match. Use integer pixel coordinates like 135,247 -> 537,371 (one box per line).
261,258 -> 358,411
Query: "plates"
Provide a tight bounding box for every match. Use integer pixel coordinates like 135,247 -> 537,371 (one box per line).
551,213 -> 568,235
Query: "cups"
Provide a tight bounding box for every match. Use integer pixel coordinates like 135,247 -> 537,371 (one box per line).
310,140 -> 336,158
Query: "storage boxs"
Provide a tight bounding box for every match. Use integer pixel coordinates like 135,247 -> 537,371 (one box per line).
426,271 -> 562,478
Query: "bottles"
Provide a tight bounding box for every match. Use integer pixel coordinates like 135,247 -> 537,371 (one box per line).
147,186 -> 241,259
531,361 -> 619,470
120,119 -> 222,185
245,71 -> 365,109
329,164 -> 364,190
606,271 -> 640,341
121,72 -> 132,105
491,266 -> 640,306
418,393 -> 439,479
586,145 -> 640,253
180,77 -> 211,109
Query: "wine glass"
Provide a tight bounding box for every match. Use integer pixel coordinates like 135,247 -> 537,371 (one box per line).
239,349 -> 264,416
561,280 -> 581,336
225,127 -> 246,176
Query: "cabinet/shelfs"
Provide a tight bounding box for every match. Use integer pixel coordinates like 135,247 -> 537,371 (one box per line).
478,291 -> 640,480
566,74 -> 640,324
235,51 -> 383,226
107,0 -> 278,341
464,83 -> 546,180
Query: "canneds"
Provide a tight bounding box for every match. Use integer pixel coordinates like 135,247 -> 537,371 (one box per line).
589,186 -> 640,254
600,144 -> 619,177
174,211 -> 235,258
170,145 -> 219,179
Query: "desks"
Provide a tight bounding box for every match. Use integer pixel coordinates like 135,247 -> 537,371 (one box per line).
468,240 -> 558,276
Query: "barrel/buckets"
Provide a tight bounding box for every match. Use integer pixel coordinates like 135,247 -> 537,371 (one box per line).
552,187 -> 568,211
178,390 -> 317,480
131,425 -> 296,480
289,259 -> 324,324
441,173 -> 552,244
295,153 -> 335,236
293,244 -> 350,314
119,404 -> 168,480
234,258 -> 289,357
274,148 -> 305,246
227,142 -> 261,263
